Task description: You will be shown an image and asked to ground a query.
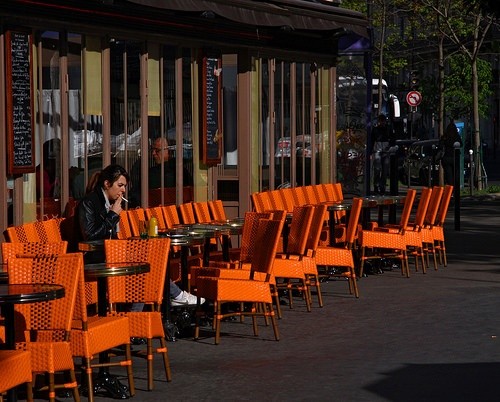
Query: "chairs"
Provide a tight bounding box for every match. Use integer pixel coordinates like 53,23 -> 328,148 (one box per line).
0,182 -> 453,402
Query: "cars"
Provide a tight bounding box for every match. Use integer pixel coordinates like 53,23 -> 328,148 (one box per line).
382,138 -> 443,187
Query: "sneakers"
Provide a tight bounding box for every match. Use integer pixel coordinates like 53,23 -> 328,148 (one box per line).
171,290 -> 206,307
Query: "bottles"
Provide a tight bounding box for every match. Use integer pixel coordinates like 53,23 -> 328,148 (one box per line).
148,216 -> 158,237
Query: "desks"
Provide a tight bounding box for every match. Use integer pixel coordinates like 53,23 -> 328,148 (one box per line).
140,230 -> 216,328
396,194 -> 422,263
210,218 -> 251,322
73,262 -> 151,399
322,202 -> 352,280
378,197 -> 408,267
80,237 -> 193,340
0,283 -> 66,402
366,198 -> 394,272
343,200 -> 377,276
279,210 -> 295,298
174,224 -> 231,323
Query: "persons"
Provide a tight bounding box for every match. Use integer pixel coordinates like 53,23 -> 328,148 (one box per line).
78,164 -> 205,312
37,139 -> 80,200
136,137 -> 192,190
372,109 -> 488,194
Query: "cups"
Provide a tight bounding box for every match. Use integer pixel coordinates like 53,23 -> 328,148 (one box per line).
139,220 -> 148,238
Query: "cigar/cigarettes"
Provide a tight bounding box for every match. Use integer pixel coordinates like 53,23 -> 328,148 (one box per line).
120,196 -> 128,202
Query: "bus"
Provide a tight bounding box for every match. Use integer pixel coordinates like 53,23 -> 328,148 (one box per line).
338,76 -> 400,177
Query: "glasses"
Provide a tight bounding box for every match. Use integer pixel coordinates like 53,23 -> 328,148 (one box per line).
151,148 -> 168,153
